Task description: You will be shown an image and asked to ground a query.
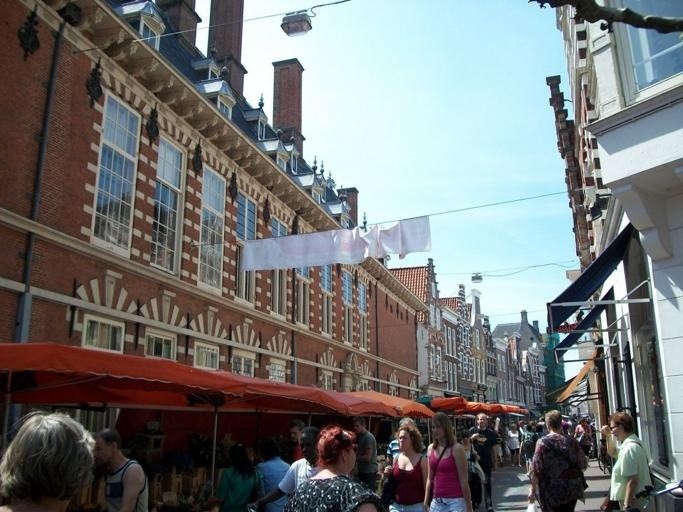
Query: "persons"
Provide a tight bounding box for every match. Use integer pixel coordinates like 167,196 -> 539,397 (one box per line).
459,429 -> 484,512
0,407 -> 96,511
384,424 -> 428,511
288,418 -> 306,463
526,410 -> 589,511
94,428 -> 149,512
468,412 -> 498,512
255,441 -> 291,511
247,426 -> 320,509
496,413 -> 596,473
207,443 -> 265,512
422,411 -> 473,511
599,412 -> 654,512
284,424 -> 383,511
352,416 -> 378,492
384,417 -> 417,468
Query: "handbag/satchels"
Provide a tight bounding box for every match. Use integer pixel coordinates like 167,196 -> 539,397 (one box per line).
380,474 -> 395,507
581,472 -> 589,490
